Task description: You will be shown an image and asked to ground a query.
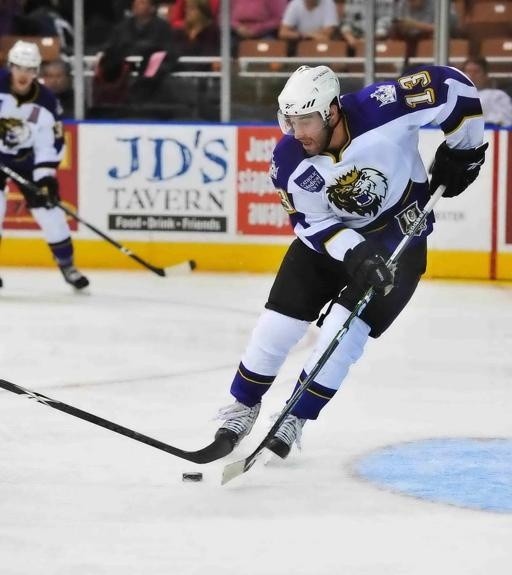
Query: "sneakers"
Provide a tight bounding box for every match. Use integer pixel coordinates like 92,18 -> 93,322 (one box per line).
59,264 -> 89,289
263,407 -> 308,458
215,400 -> 261,446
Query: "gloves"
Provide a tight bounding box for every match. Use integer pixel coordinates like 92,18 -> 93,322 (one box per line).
427,141 -> 489,199
344,243 -> 399,303
32,176 -> 60,208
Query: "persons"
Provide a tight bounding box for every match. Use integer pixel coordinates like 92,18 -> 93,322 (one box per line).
0,39 -> 91,291
215,63 -> 488,459
460,56 -> 511,127
0,0 -> 239,122
228,0 -> 458,56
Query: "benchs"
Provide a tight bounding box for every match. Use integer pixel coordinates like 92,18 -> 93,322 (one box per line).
0,0 -> 512,118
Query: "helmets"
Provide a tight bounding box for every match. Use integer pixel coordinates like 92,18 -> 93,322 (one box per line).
7,41 -> 43,72
275,66 -> 342,139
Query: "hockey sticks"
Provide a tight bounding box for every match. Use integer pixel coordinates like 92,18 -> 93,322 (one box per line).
1,379 -> 234,464
221,185 -> 445,485
0,161 -> 196,276
